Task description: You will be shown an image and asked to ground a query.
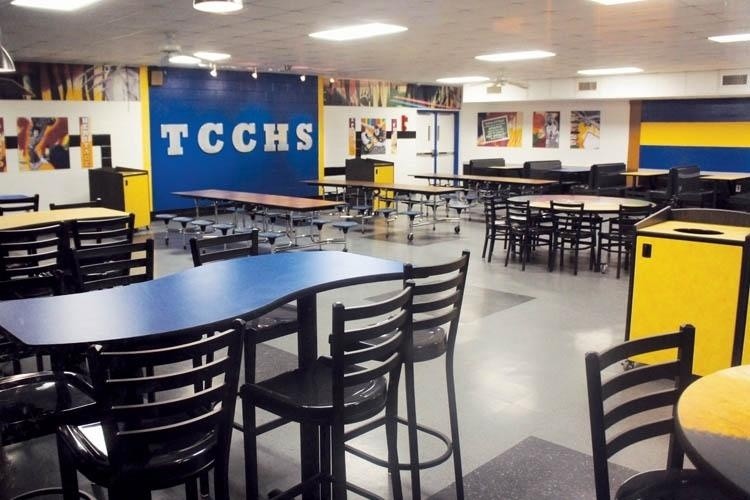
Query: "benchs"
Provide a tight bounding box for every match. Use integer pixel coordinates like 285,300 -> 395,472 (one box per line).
411,152 -> 749,212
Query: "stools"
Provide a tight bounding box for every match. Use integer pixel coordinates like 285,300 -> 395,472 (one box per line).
1,180 -> 468,500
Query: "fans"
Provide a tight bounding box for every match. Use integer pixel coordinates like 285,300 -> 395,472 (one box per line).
471,67 -> 530,94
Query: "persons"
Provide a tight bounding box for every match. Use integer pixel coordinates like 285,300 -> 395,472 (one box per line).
326,83 -> 339,102
575,112 -> 591,148
545,114 -> 559,147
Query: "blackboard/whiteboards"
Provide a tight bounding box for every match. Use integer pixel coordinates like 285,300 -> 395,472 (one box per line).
481,116 -> 510,144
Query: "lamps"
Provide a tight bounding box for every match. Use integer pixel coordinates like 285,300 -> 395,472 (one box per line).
208,64 -> 337,83
0,43 -> 17,73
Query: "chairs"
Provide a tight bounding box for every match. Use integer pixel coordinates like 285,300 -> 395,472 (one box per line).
579,322 -> 739,497
482,211 -> 658,280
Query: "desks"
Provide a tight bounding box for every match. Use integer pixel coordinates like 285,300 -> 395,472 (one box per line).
670,365 -> 750,495
